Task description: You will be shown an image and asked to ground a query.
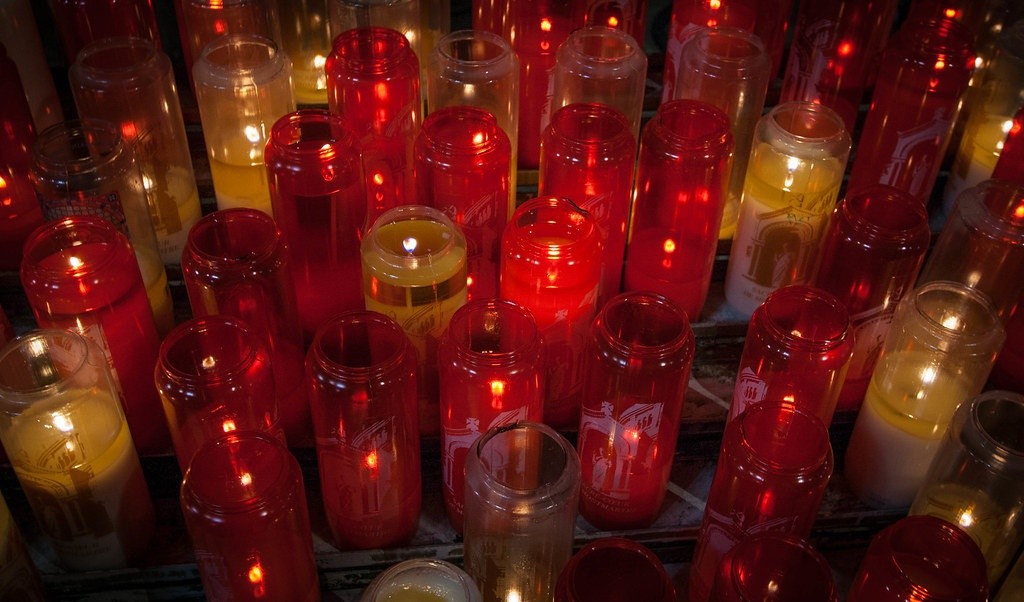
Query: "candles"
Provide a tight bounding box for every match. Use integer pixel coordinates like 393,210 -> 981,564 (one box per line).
0,0 -> 1024,602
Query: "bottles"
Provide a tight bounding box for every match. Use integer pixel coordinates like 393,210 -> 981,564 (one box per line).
0,2 -> 1022,599
